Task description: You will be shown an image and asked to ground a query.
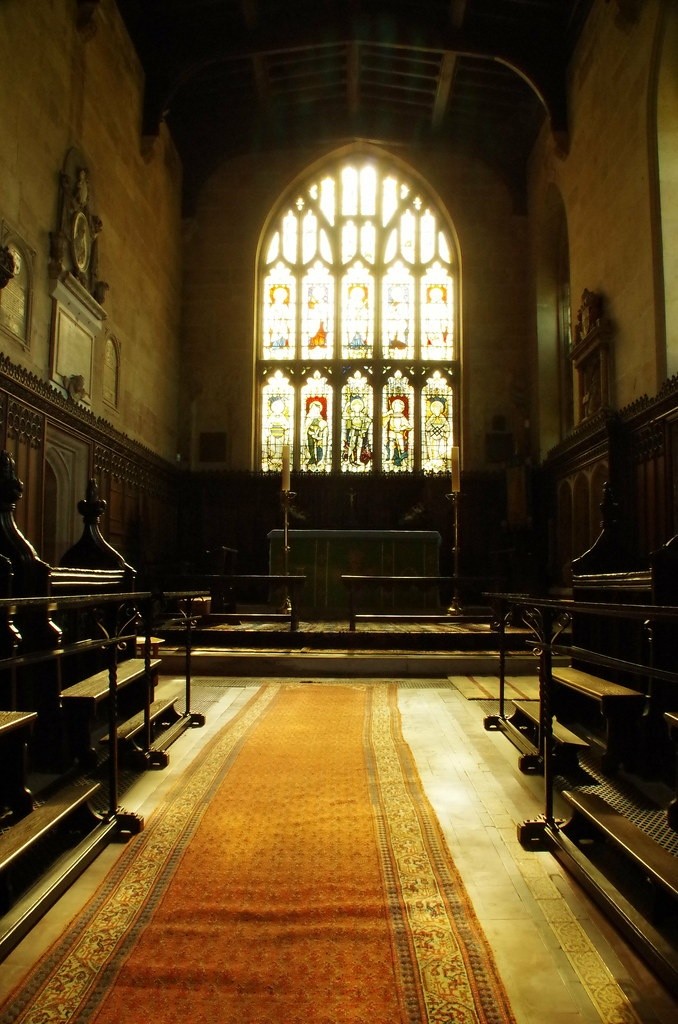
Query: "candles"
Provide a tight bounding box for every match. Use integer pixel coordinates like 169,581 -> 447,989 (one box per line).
451,447 -> 460,492
282,445 -> 290,491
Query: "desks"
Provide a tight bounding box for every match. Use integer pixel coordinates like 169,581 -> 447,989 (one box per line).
147,574 -> 308,630
340,574 -> 509,632
